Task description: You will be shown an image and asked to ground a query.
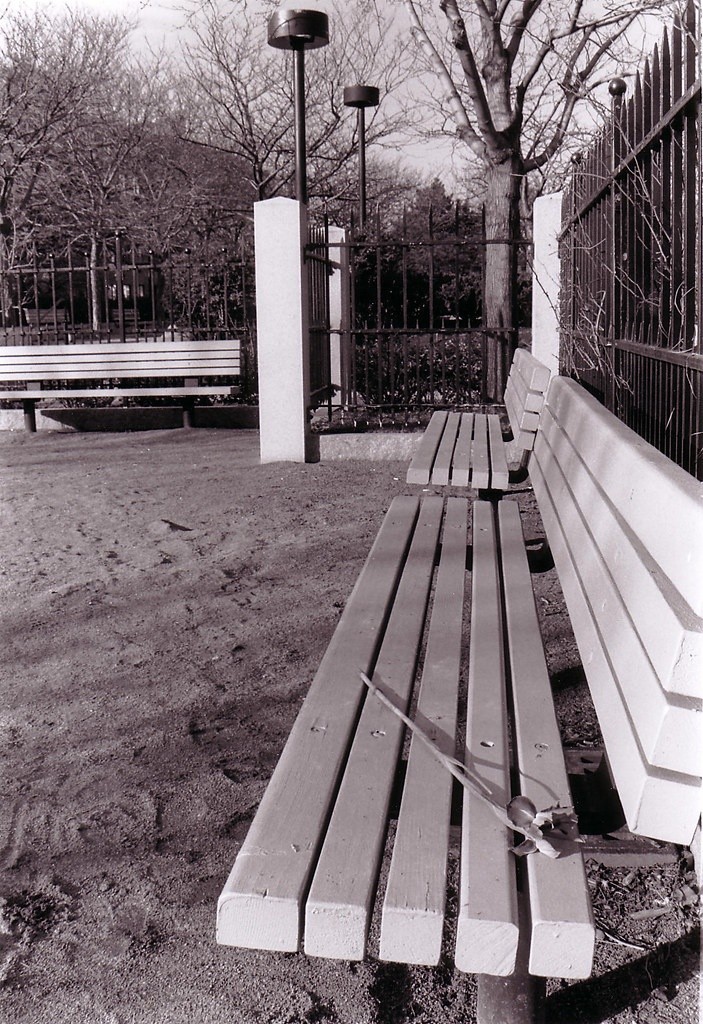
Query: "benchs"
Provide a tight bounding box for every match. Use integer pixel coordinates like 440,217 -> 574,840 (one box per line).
0,340 -> 245,433
217,376 -> 703,1024
406,348 -> 552,549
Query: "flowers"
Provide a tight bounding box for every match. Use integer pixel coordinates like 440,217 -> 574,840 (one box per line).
358,667 -> 579,859
